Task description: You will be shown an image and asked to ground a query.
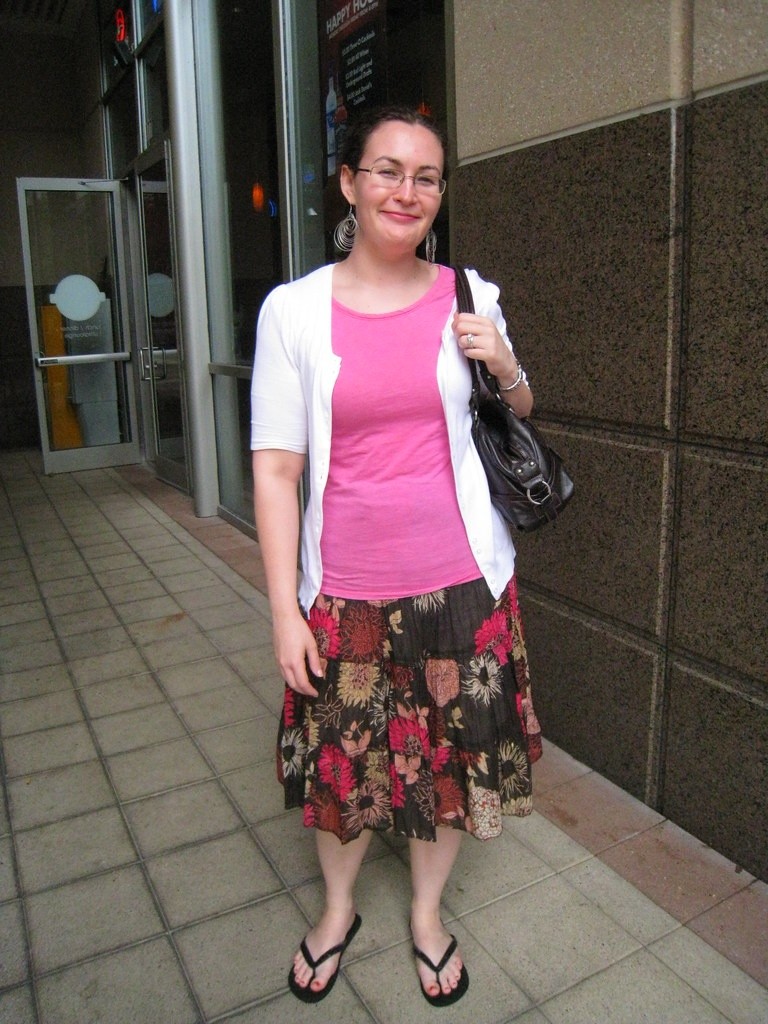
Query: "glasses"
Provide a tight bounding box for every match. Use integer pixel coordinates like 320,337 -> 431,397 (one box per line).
353,167 -> 448,197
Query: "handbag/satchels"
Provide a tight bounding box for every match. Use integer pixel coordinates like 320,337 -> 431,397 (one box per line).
451,262 -> 575,533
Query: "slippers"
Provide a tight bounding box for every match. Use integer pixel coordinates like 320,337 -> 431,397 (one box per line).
408,915 -> 470,1006
288,912 -> 361,1005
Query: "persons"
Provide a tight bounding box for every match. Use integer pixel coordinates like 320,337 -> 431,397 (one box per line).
251,104 -> 535,1007
